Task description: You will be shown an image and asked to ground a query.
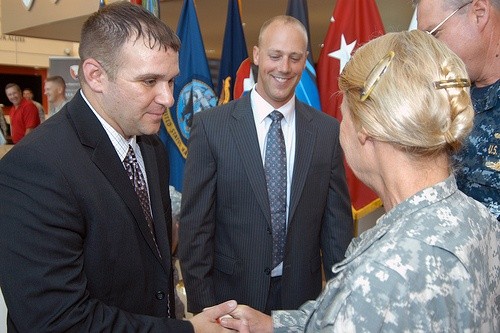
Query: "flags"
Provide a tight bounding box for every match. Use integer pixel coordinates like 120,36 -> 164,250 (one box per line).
100,0 -> 389,221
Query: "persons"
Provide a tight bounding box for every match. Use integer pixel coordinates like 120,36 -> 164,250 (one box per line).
413,0 -> 500,223
0,75 -> 68,144
0,0 -> 239,333
178,15 -> 355,317
200,30 -> 500,333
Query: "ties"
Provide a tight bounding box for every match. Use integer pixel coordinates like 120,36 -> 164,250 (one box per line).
263,111 -> 287,270
124,145 -> 171,319
